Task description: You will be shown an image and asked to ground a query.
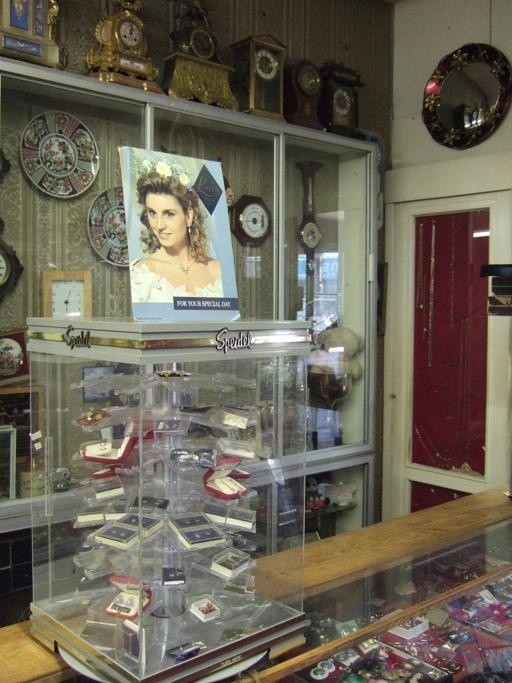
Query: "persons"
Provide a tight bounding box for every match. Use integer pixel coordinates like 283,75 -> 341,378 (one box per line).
124,154 -> 226,306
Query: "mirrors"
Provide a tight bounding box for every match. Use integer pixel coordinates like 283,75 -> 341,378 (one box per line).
423,43 -> 512,150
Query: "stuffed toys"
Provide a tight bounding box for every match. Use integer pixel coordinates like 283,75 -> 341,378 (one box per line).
302,323 -> 365,383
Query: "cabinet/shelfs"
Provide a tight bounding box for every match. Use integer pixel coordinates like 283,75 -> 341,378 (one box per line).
0,55 -> 381,624
0,488 -> 512,683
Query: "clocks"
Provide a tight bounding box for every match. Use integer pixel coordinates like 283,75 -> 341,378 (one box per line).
83,0 -> 165,96
296,160 -> 325,322
229,35 -> 288,123
162,0 -> 239,112
284,60 -> 326,131
320,61 -> 366,139
0,218 -> 23,304
0,0 -> 68,71
231,194 -> 272,247
453,104 -> 472,129
41,270 -> 92,318
